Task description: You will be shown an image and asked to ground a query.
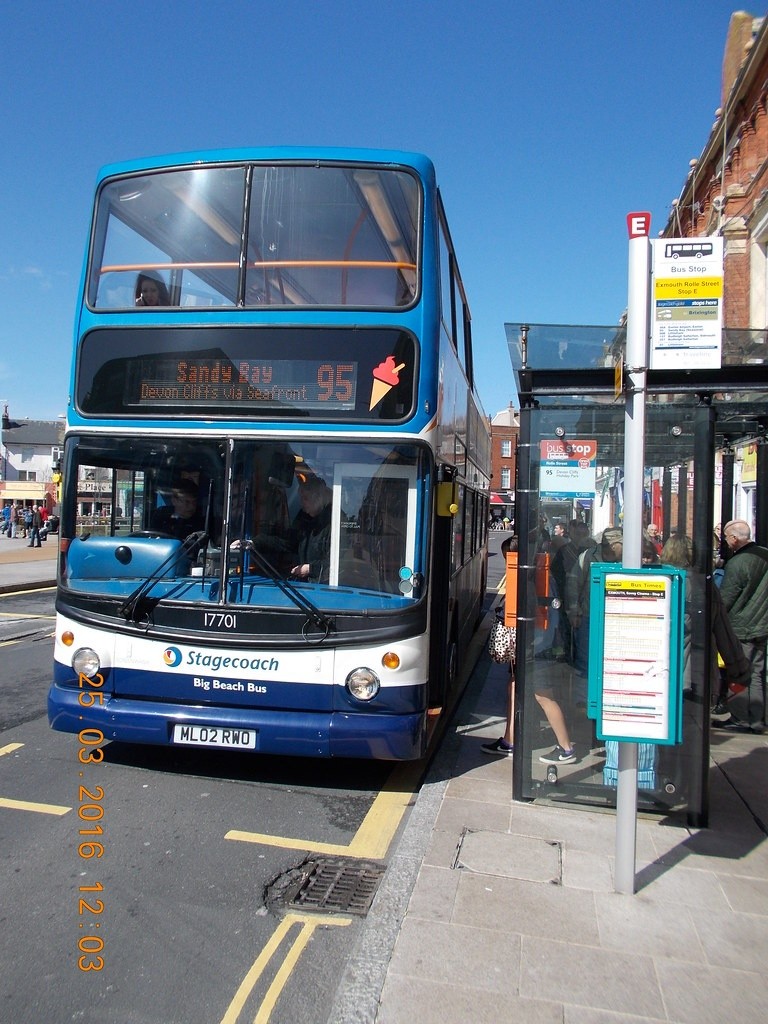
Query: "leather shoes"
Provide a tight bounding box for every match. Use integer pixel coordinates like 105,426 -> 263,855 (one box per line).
752,726 -> 766,734
712,719 -> 751,733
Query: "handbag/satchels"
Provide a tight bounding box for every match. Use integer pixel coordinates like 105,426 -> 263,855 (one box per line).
488,620 -> 517,664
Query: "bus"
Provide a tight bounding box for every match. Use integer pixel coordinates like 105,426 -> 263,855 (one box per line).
45,143 -> 494,761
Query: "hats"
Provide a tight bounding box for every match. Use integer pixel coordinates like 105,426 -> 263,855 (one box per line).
602,528 -> 623,544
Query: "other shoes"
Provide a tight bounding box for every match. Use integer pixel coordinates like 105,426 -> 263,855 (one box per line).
28,545 -> 34,547
35,545 -> 41,547
712,705 -> 728,714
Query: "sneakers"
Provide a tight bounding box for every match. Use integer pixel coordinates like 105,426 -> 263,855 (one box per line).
539,745 -> 577,765
480,737 -> 513,756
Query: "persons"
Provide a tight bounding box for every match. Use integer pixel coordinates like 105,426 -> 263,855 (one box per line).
479,519 -> 766,765
135,269 -> 171,306
139,456 -> 381,589
2,501 -> 60,549
99,503 -> 122,530
